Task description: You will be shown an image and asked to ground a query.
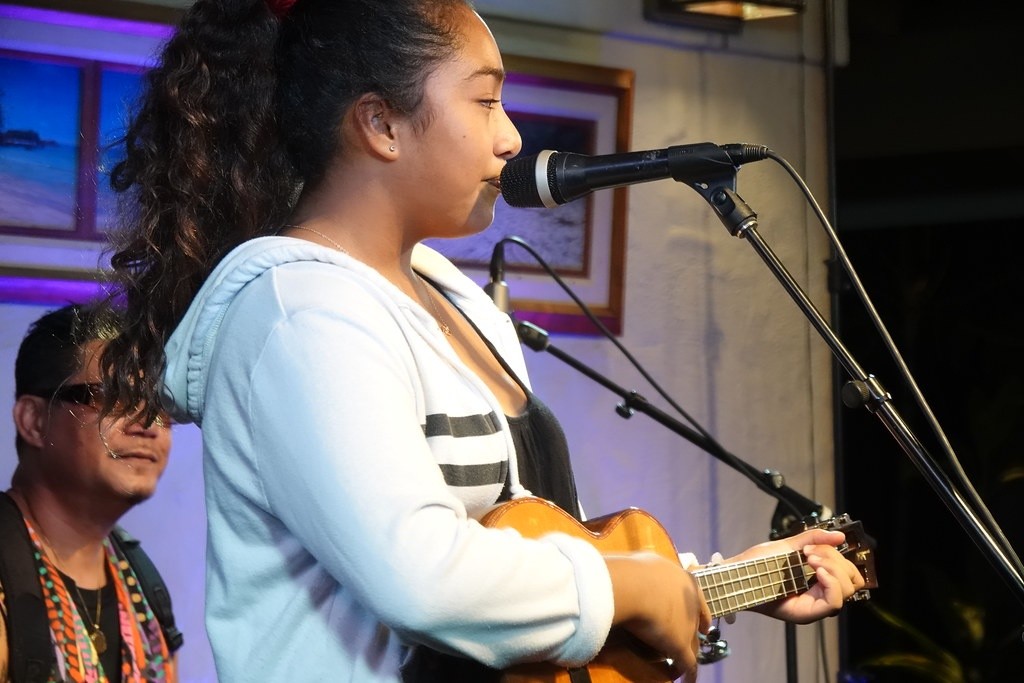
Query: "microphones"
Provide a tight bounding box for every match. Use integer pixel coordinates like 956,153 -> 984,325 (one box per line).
499,143 -> 769,210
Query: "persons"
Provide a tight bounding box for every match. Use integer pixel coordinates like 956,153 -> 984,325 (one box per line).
0,303 -> 190,682
98,1 -> 865,683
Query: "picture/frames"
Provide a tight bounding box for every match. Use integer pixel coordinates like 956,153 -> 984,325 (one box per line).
413,54 -> 643,340
0,2 -> 205,314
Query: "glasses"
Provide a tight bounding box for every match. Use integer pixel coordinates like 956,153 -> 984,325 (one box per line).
46,383 -> 177,424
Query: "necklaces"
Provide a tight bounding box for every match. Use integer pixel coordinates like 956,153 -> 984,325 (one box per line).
289,224 -> 452,336
37,528 -> 107,655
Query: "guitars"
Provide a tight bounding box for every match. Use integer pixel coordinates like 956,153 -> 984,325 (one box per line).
462,498 -> 884,683
